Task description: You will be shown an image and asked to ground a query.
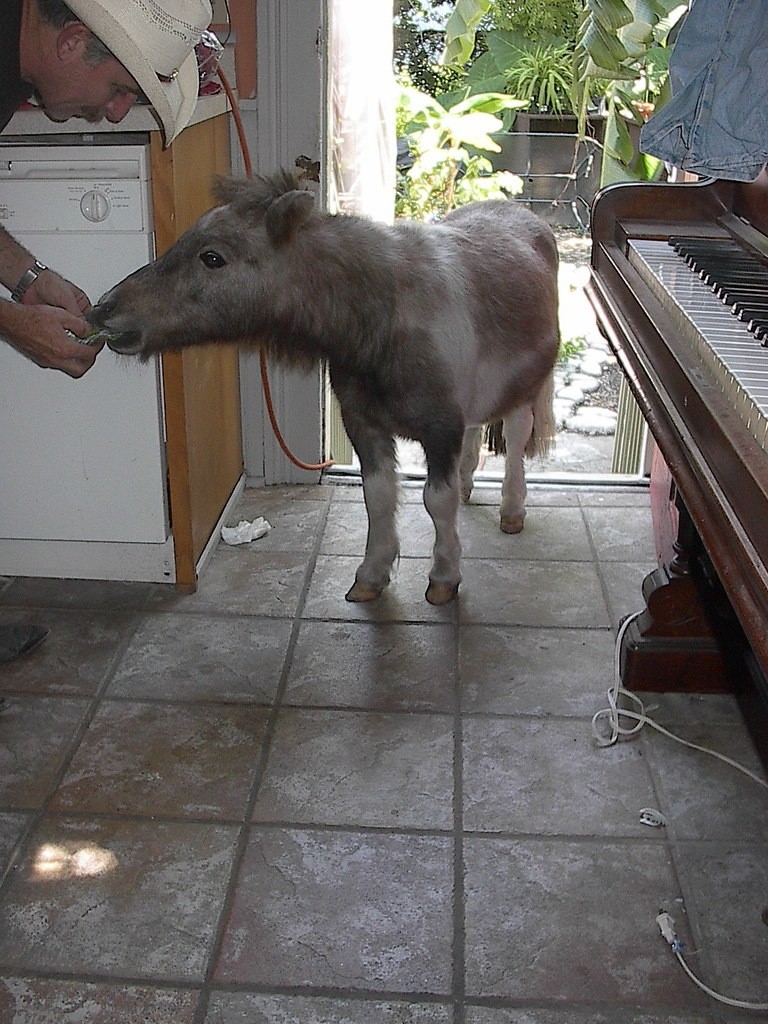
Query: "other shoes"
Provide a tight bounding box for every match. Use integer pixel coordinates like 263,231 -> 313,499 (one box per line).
0,623 -> 51,664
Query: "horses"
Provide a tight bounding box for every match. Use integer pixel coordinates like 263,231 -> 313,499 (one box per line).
85,166 -> 565,605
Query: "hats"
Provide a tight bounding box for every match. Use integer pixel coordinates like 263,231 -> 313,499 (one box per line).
63,0 -> 213,152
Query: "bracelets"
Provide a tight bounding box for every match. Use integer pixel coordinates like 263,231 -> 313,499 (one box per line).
10,261 -> 48,303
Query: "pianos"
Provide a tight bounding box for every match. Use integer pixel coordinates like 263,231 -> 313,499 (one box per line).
581,155 -> 766,772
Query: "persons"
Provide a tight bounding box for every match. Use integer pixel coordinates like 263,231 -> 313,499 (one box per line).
0,0 -> 211,663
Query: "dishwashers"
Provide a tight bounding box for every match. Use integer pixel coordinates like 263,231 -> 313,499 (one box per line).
0,144 -> 172,543
